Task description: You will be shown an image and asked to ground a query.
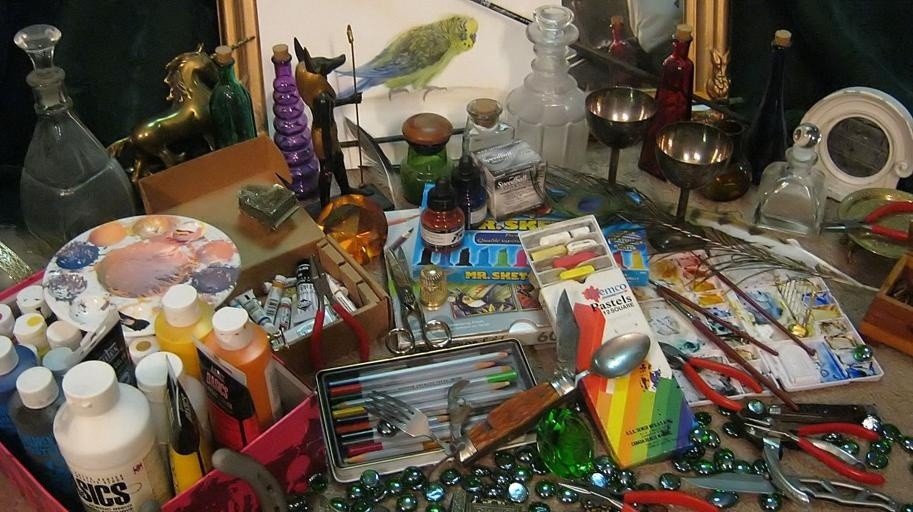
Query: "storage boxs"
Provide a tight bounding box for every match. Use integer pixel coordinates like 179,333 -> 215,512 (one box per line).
1,269 -> 328,512
138,136 -> 396,359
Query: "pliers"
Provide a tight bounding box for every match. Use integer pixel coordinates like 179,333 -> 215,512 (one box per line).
307,255 -> 367,376
552,482 -> 717,512
659,340 -> 881,503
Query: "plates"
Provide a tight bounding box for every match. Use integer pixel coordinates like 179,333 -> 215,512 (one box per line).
836,186 -> 912,260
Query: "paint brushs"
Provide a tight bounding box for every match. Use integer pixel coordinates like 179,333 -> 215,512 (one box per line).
646,251 -> 815,413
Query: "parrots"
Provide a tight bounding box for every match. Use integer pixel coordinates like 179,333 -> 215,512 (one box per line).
335,13 -> 479,101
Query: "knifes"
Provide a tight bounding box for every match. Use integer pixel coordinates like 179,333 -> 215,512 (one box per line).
678,472 -> 903,511
554,288 -> 580,379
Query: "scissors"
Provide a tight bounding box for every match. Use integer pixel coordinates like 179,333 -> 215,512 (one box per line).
384,247 -> 452,357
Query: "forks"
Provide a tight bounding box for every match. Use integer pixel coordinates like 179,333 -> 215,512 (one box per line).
361,389 -> 452,461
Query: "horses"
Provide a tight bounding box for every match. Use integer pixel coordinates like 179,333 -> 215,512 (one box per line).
105,44 -> 218,196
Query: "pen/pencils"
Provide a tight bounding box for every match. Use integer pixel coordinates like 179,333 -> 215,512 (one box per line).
328,352 -> 526,465
385,213 -> 423,251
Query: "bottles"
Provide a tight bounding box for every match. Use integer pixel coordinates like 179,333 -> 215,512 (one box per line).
504,4 -> 592,173
749,121 -> 830,242
270,43 -> 321,202
739,25 -> 796,186
658,24 -> 697,122
398,111 -> 456,207
12,16 -> 139,263
605,12 -> 641,85
208,44 -> 258,154
460,97 -> 515,165
699,119 -> 754,203
419,162 -> 489,253
1,284 -> 287,512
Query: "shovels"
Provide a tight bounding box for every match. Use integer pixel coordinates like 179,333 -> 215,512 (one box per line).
825,198 -> 911,245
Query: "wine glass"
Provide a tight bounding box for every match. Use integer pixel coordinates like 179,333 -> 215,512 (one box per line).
583,85 -> 659,206
647,118 -> 733,252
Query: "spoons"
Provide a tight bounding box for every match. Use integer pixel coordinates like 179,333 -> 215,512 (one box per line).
574,330 -> 651,387
